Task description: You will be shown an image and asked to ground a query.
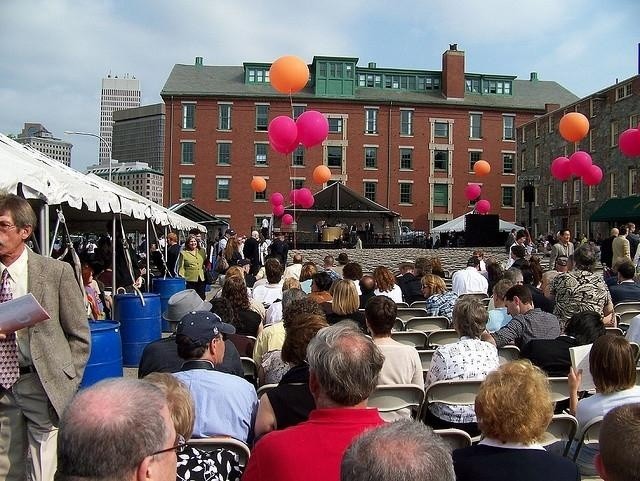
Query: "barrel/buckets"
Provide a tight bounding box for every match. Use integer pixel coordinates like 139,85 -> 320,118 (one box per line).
114,293 -> 162,368
75,320 -> 123,394
152,278 -> 185,332
211,255 -> 216,276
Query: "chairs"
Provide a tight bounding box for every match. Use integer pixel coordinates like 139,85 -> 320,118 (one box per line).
185,266 -> 639,481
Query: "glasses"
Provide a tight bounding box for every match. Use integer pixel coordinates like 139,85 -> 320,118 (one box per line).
147,432 -> 187,457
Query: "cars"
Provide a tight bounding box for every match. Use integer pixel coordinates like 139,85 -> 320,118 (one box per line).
159,236 -> 169,248
47,231 -> 107,255
401,226 -> 427,244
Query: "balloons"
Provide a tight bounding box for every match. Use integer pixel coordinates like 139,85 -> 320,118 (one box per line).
464,160 -> 492,215
550,111 -> 603,186
249,55 -> 332,228
618,128 -> 640,157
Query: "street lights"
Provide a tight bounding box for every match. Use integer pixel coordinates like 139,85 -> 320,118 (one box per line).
518,172 -> 541,238
62,128 -> 113,184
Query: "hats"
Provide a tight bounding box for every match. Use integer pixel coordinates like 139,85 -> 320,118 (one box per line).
398,258 -> 417,270
175,310 -> 236,350
335,253 -> 351,264
